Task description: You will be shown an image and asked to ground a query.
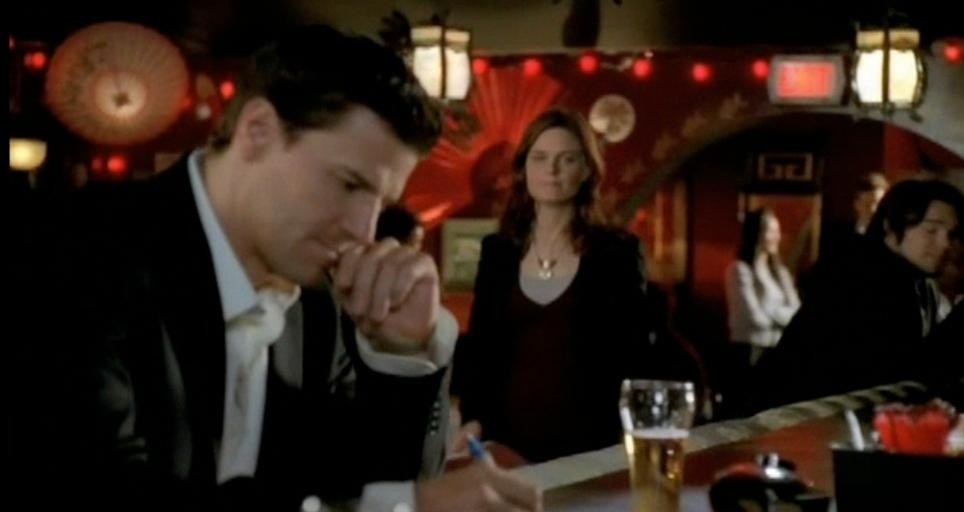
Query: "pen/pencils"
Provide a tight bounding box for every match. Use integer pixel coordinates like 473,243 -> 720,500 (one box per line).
466,432 -> 496,464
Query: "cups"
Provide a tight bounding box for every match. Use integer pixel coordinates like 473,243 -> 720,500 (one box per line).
621,379 -> 694,510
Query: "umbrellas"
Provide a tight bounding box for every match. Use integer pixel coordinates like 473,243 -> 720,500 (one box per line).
404,66 -> 573,232
40,17 -> 194,150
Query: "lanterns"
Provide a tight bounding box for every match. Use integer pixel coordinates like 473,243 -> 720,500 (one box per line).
376,12 -> 482,139
845,21 -> 931,123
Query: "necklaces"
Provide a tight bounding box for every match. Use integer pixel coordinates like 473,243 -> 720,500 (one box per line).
530,232 -> 572,283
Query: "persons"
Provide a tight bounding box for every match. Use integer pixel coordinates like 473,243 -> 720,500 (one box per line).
707,178 -> 963,426
447,104 -> 655,475
723,204 -> 804,391
0,24 -> 547,512
847,170 -> 891,236
374,202 -> 428,250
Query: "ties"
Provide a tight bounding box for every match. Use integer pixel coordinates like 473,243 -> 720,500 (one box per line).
218,309 -> 268,489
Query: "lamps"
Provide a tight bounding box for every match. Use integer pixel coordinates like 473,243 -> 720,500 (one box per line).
410,1 -> 470,109
849,1 -> 928,126
